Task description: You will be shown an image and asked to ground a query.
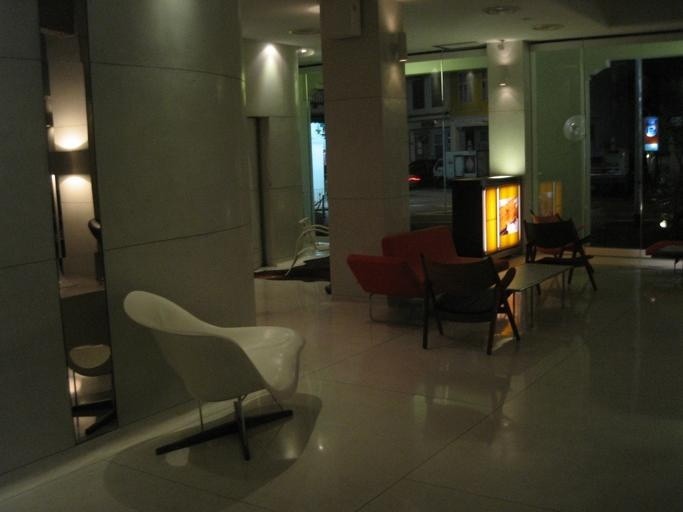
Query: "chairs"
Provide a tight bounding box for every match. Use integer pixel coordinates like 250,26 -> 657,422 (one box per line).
520,211 -> 601,296
346,252 -> 521,356
124,287 -> 324,472
254,223 -> 330,281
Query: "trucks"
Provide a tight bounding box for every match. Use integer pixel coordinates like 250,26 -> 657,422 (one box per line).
409,150 -> 481,206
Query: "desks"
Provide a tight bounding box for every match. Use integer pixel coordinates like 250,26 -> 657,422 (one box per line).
493,263 -> 577,331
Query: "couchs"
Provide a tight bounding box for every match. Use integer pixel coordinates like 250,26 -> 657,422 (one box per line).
382,224 -> 510,309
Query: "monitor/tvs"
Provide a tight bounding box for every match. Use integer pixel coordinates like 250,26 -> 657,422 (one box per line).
452,174 -> 524,259
